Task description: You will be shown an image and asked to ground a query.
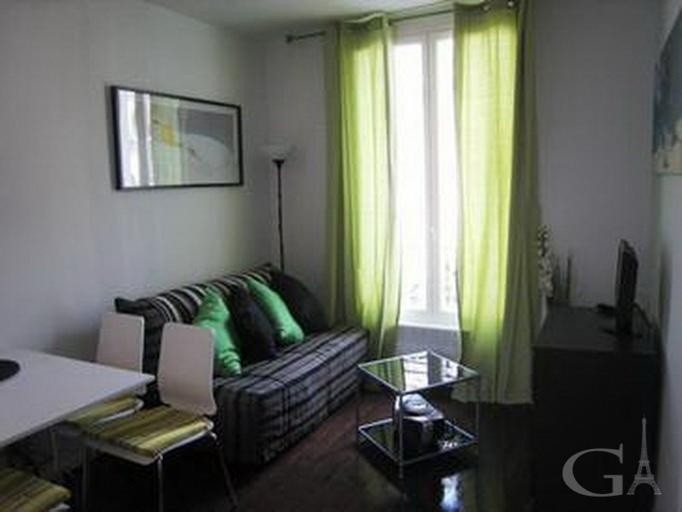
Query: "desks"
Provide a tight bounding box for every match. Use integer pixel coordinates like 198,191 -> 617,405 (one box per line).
529,301 -> 664,502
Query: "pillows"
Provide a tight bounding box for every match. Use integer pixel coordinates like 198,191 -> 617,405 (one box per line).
188,289 -> 241,376
269,267 -> 331,333
226,283 -> 278,362
241,276 -> 309,353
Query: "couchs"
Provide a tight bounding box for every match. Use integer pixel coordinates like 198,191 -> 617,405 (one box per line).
112,262 -> 372,464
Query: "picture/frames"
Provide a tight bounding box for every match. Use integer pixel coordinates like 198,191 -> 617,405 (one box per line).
108,84 -> 246,191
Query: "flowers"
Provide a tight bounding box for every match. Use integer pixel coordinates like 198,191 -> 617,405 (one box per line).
534,226 -> 572,316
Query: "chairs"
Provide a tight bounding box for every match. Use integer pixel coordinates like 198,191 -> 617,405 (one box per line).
79,321 -> 247,509
47,311 -> 147,508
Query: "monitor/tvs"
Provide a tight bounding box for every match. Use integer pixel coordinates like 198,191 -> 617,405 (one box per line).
613,238 -> 638,337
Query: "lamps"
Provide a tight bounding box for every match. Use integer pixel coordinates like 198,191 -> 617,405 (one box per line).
264,144 -> 294,276
